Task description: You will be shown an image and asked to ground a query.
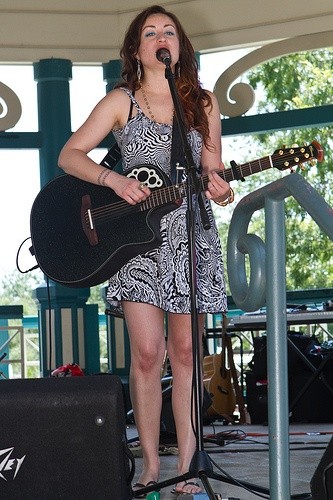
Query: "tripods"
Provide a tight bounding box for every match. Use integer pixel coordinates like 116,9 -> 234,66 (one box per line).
131,160 -> 270,500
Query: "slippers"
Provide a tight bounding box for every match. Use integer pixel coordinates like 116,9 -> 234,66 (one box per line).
170,480 -> 204,495
131,479 -> 161,499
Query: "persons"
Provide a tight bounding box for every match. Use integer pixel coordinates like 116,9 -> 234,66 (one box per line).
56,6 -> 230,496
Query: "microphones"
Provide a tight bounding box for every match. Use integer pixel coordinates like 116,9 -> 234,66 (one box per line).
157,49 -> 172,65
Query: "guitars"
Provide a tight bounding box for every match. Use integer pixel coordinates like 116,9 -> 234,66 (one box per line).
202,311 -> 236,419
30,141 -> 323,288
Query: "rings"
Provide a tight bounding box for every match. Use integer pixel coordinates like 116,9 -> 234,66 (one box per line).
138,183 -> 146,190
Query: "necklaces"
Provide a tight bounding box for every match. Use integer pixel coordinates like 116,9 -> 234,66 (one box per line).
140,81 -> 174,141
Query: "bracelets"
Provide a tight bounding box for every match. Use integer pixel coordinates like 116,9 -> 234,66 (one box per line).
97,168 -> 110,186
102,170 -> 113,187
213,187 -> 234,207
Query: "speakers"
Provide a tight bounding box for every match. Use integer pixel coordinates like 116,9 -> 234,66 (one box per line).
309,435 -> 333,500
0,374 -> 130,500
247,330 -> 333,425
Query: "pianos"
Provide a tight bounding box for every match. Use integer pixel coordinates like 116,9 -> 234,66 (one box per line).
234,311 -> 333,326
